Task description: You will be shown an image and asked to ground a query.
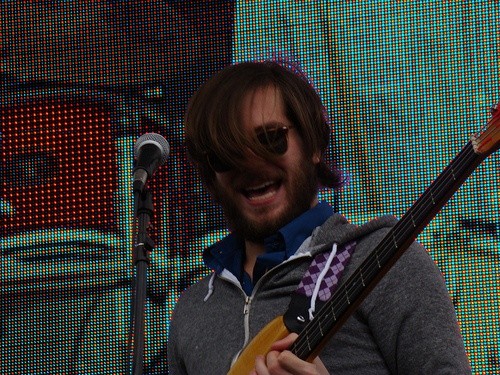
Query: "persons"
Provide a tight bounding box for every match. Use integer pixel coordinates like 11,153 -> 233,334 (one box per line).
164,61 -> 475,375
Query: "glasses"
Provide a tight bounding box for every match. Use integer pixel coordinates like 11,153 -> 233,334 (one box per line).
206,125 -> 298,174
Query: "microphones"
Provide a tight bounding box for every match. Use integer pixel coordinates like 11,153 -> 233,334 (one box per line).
131,132 -> 171,191
